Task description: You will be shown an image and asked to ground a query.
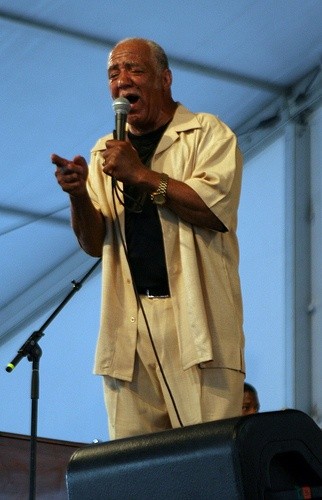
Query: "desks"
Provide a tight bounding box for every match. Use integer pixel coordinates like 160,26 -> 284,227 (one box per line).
0,431 -> 90,500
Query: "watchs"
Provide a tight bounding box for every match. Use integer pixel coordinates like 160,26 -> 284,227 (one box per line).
151,173 -> 168,207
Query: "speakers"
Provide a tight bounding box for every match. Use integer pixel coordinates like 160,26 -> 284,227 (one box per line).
66,408 -> 322,500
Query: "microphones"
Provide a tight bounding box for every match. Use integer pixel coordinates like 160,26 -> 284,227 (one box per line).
112,97 -> 131,141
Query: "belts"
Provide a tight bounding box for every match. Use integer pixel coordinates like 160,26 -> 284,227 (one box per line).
134,280 -> 170,298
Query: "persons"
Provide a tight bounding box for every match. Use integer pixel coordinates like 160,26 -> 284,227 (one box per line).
241,382 -> 260,416
52,38 -> 245,442
